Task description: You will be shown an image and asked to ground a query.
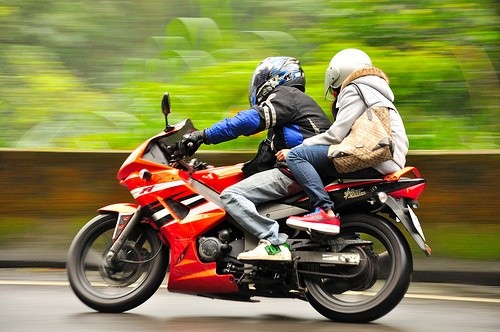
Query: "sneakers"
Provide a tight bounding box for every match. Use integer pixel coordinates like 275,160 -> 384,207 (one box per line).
286,208 -> 340,234
238,239 -> 292,261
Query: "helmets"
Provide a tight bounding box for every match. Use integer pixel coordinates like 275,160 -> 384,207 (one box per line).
325,48 -> 373,99
248,56 -> 306,108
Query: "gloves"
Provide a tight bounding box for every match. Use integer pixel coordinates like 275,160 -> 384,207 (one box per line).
181,130 -> 205,155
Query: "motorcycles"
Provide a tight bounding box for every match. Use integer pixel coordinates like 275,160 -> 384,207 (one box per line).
67,92 -> 433,324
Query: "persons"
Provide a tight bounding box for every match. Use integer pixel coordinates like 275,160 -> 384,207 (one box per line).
275,47 -> 410,235
179,55 -> 332,262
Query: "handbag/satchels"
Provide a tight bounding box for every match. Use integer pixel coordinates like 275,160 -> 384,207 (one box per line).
242,141 -> 279,175
327,82 -> 394,174
